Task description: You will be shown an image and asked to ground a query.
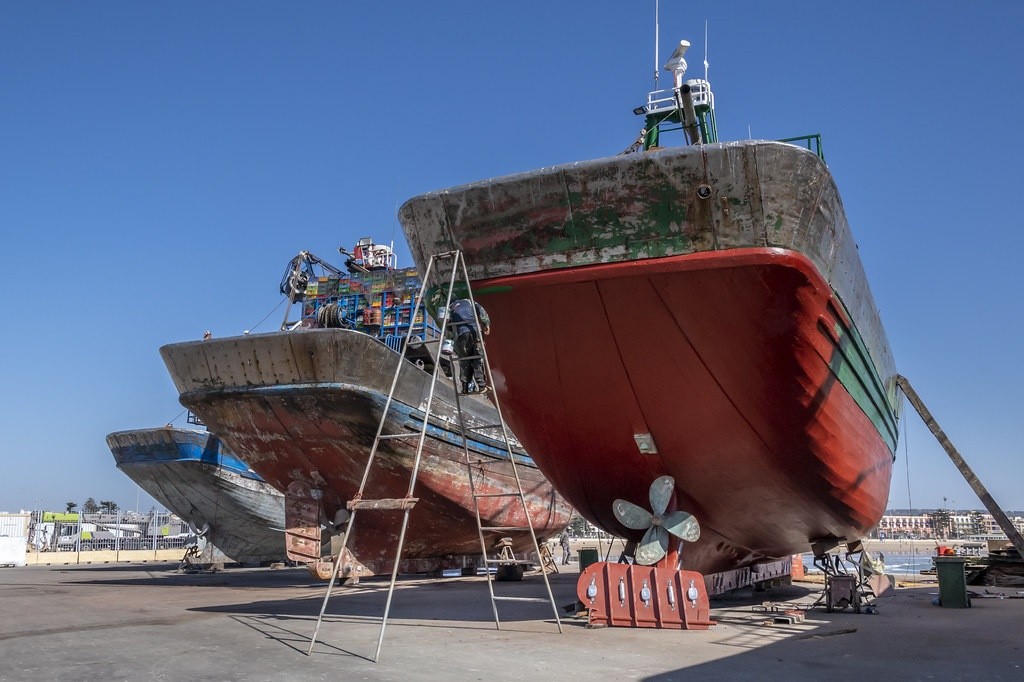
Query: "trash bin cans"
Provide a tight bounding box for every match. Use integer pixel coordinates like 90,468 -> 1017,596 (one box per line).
577,547 -> 598,573
931,556 -> 972,608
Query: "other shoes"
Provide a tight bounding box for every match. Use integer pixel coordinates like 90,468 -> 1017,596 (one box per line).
480,386 -> 488,394
565,562 -> 570,565
461,389 -> 468,395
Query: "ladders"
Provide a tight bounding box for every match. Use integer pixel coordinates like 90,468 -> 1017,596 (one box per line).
306,251 -> 563,662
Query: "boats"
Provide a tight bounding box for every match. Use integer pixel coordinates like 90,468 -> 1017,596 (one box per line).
104,409 -> 332,568
157,237 -> 571,576
398,2 -> 903,576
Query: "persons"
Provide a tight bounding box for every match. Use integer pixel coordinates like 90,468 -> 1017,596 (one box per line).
560,526 -> 572,565
447,299 -> 492,394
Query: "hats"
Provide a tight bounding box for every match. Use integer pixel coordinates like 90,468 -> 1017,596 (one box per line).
567,527 -> 572,530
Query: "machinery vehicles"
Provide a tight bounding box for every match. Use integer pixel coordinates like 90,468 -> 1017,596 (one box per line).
27,511 -> 169,552
